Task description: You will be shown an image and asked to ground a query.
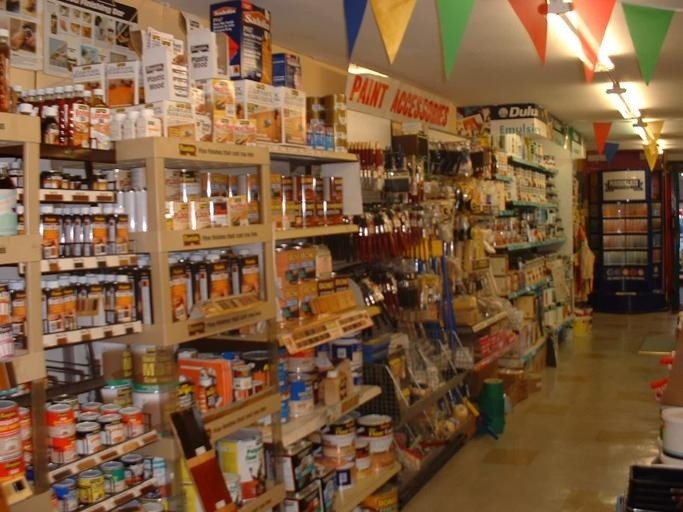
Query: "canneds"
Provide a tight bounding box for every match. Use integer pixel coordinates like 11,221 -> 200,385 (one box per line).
46,394 -> 145,512
270,174 -> 343,228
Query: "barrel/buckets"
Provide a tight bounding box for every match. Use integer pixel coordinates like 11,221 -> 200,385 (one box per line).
215,428 -> 266,499
362,482 -> 399,512
661,407 -> 683,460
321,415 -> 356,465
357,413 -> 396,466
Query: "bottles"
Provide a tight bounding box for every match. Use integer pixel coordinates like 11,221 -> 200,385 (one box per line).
0,251 -> 262,358
112,107 -> 160,142
0,160 -> 129,261
286,343 -> 334,418
0,28 -> 11,113
12,85 -> 114,152
